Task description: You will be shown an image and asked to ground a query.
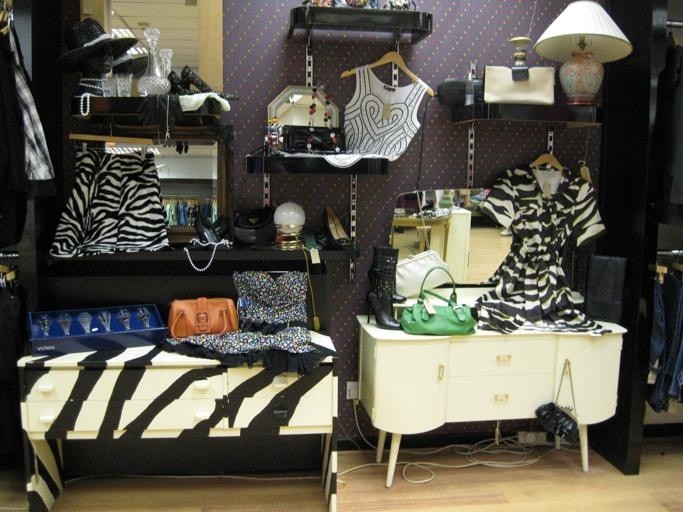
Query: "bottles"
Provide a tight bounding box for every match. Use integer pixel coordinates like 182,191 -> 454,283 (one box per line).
136,27 -> 171,95
464,60 -> 478,106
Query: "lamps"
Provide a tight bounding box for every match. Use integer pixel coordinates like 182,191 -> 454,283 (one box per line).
533,0 -> 635,107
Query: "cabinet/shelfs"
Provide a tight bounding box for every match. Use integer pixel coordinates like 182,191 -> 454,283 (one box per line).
599,1 -> 683,476
17,332 -> 340,512
356,313 -> 628,489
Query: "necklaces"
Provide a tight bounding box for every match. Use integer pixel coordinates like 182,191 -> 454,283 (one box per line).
184,239 -> 231,271
73,76 -> 106,118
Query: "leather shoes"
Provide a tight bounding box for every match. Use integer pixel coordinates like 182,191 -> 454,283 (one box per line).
316,205 -> 353,251
188,214 -> 232,249
169,64 -> 240,100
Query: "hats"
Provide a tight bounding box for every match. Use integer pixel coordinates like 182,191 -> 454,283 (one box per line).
45,20 -> 138,77
110,52 -> 150,73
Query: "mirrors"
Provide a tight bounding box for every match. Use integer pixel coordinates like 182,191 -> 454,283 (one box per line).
104,0 -> 197,78
105,142 -> 217,228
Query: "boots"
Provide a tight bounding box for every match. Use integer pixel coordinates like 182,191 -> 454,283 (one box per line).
368,246 -> 405,303
367,270 -> 403,329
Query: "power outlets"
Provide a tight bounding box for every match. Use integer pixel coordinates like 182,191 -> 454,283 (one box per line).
346,380 -> 360,400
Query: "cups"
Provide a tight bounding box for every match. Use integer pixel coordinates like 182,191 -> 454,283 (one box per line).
100,73 -> 116,98
114,74 -> 132,97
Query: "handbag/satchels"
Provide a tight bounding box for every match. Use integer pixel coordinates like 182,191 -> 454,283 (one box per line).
235,207 -> 277,245
169,298 -> 237,339
396,250 -> 453,299
535,403 -> 579,444
305,0 -> 412,10
401,302 -> 476,335
483,66 -> 555,106
283,125 -> 346,155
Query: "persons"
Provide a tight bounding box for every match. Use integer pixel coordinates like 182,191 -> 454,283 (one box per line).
53,16 -> 139,98
112,53 -> 134,78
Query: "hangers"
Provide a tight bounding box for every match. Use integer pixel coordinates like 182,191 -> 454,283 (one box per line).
667,20 -> 679,47
341,40 -> 436,97
529,149 -> 563,170
